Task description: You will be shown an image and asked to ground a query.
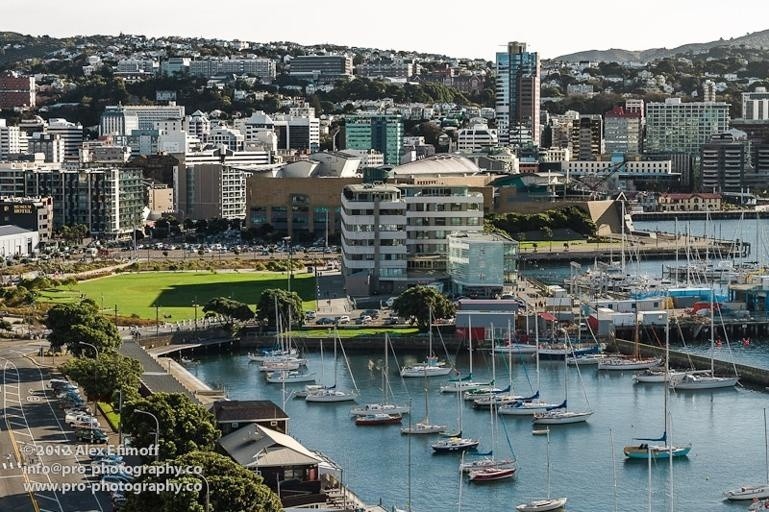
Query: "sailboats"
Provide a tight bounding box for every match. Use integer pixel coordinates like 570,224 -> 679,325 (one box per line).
392,261 -> 769,512
247,290 -> 416,428
560,195 -> 769,309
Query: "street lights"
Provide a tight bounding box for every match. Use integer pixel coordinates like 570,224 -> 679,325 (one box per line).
518,255 -> 545,284
131,407 -> 160,467
172,467 -> 211,512
78,340 -> 99,420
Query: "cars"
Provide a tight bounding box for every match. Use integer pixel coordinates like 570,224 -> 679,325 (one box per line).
301,307 -> 457,326
20,234 -> 337,263
45,374 -> 138,512
